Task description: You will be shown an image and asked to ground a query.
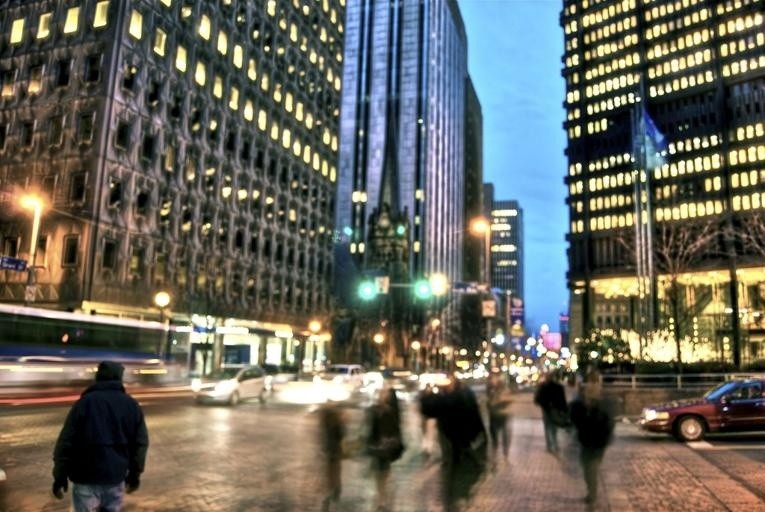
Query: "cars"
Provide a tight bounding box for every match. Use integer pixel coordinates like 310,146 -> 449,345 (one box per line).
634,379 -> 765,443
312,363 -> 368,389
193,363 -> 272,407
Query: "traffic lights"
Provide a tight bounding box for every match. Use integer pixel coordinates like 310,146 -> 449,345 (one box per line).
358,281 -> 377,301
414,272 -> 448,299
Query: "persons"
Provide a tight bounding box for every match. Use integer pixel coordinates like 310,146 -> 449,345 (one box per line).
533,373 -> 567,454
485,382 -> 512,464
52,361 -> 150,510
739,386 -> 761,399
566,370 -> 616,512
308,368 -> 488,511
555,370 -> 575,387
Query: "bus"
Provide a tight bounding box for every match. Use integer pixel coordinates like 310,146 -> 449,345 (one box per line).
0,304 -> 168,398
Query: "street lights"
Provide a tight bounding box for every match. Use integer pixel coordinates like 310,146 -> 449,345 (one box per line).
155,292 -> 170,356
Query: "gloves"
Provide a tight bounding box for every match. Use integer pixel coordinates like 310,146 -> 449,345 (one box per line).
52,478 -> 68,499
124,471 -> 140,493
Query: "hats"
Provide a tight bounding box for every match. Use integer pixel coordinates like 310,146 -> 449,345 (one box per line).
96,360 -> 123,383
